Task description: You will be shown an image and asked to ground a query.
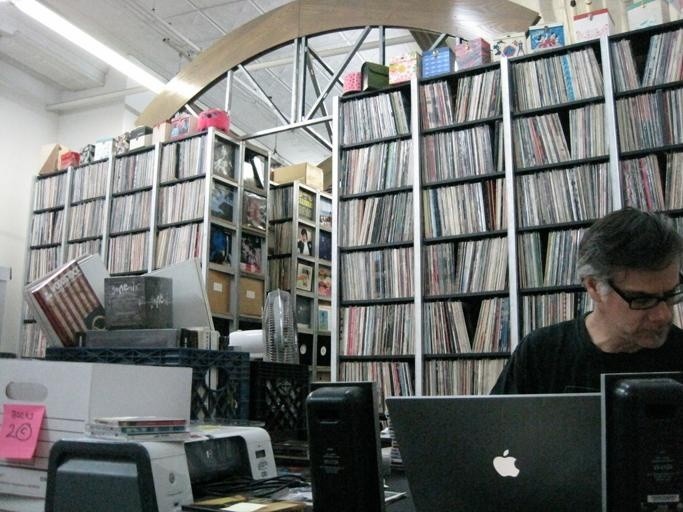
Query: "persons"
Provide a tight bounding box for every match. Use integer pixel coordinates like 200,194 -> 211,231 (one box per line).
484,206 -> 683,392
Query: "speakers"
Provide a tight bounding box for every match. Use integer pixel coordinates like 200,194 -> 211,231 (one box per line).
599,369 -> 682,511
298,380 -> 388,512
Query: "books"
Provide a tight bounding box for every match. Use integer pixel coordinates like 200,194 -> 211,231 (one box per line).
24,245 -> 108,360
84,415 -> 193,444
421,357 -> 516,397
417,72 -> 504,128
70,160 -> 110,203
29,209 -> 66,248
66,199 -> 107,241
338,138 -> 416,195
161,137 -> 236,182
319,229 -> 332,261
514,160 -> 611,229
319,197 -> 333,227
336,190 -> 415,246
338,89 -> 413,146
667,285 -> 683,325
515,226 -> 606,287
112,149 -> 155,192
296,295 -> 314,331
317,300 -> 332,333
240,231 -> 262,275
264,220 -> 315,260
517,289 -> 596,340
339,361 -> 413,415
418,124 -> 498,178
614,87 -> 683,153
317,263 -> 333,298
418,237 -> 510,296
661,215 -> 683,234
266,254 -> 315,294
617,153 -> 683,212
103,275 -> 173,331
207,269 -> 233,315
508,47 -> 611,112
610,27 -> 683,95
242,189 -> 268,231
511,101 -> 614,169
340,246 -> 411,298
31,172 -> 68,209
106,231 -> 148,274
419,178 -> 511,237
267,184 -> 315,222
339,303 -> 417,358
238,277 -> 265,316
111,190 -> 150,234
153,223 -> 235,273
419,297 -> 512,354
158,179 -> 236,225
180,323 -> 222,423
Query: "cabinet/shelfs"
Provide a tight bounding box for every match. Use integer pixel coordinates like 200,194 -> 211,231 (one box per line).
102,142 -> 156,277
414,59 -> 513,397
59,153 -> 110,271
152,127 -> 268,338
17,167 -> 67,360
506,36 -> 612,348
269,180 -> 330,383
333,78 -> 418,420
608,19 -> 683,333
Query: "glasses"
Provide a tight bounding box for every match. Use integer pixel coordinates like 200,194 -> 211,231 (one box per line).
605,272 -> 683,309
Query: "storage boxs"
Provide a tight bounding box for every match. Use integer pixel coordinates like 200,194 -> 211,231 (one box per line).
316,155 -> 331,190
273,162 -> 323,192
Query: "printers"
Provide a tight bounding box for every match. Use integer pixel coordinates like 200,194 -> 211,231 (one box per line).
43,422 -> 280,512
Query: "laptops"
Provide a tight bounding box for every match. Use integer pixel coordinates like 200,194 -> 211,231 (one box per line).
383,393 -> 604,509
